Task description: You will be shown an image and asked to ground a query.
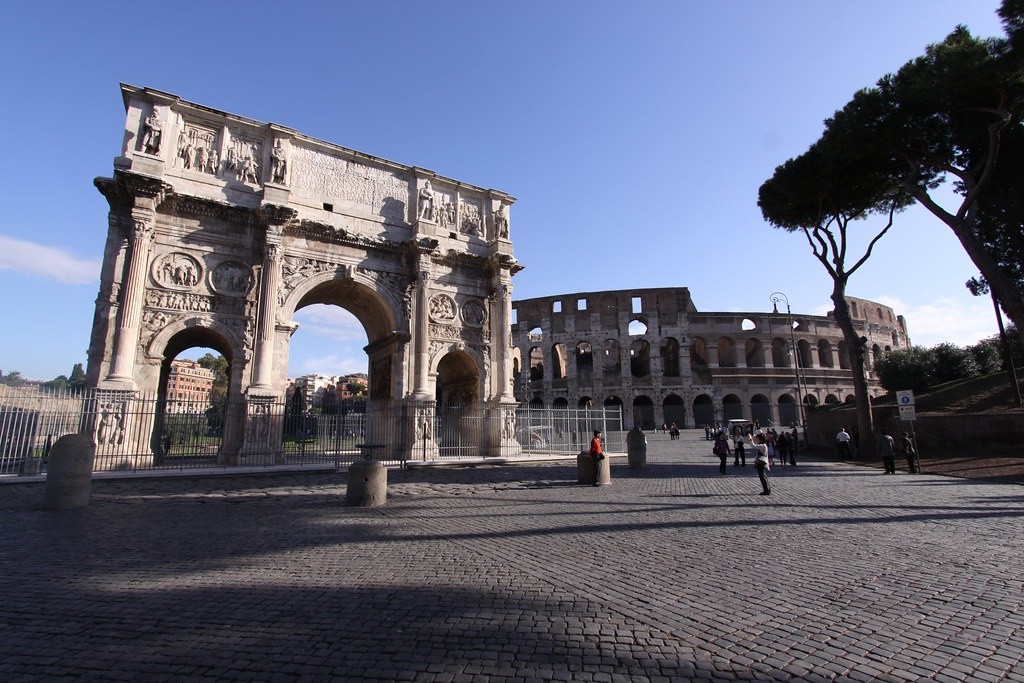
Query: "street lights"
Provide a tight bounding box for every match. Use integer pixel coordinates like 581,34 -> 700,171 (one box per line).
768,291 -> 810,451
786,340 -> 810,405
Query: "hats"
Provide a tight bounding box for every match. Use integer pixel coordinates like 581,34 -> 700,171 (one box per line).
593,429 -> 601,437
758,429 -> 763,434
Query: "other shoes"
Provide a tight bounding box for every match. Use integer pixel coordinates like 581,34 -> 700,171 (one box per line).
759,492 -> 771,495
593,481 -> 602,487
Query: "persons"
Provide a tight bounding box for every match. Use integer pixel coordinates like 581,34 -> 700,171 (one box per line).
590,429 -> 603,487
558,424 -> 578,446
902,432 -> 918,475
877,429 -> 896,474
662,419 -> 860,495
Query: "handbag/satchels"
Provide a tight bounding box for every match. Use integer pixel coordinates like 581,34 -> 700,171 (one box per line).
599,453 -> 605,459
713,447 -> 718,454
